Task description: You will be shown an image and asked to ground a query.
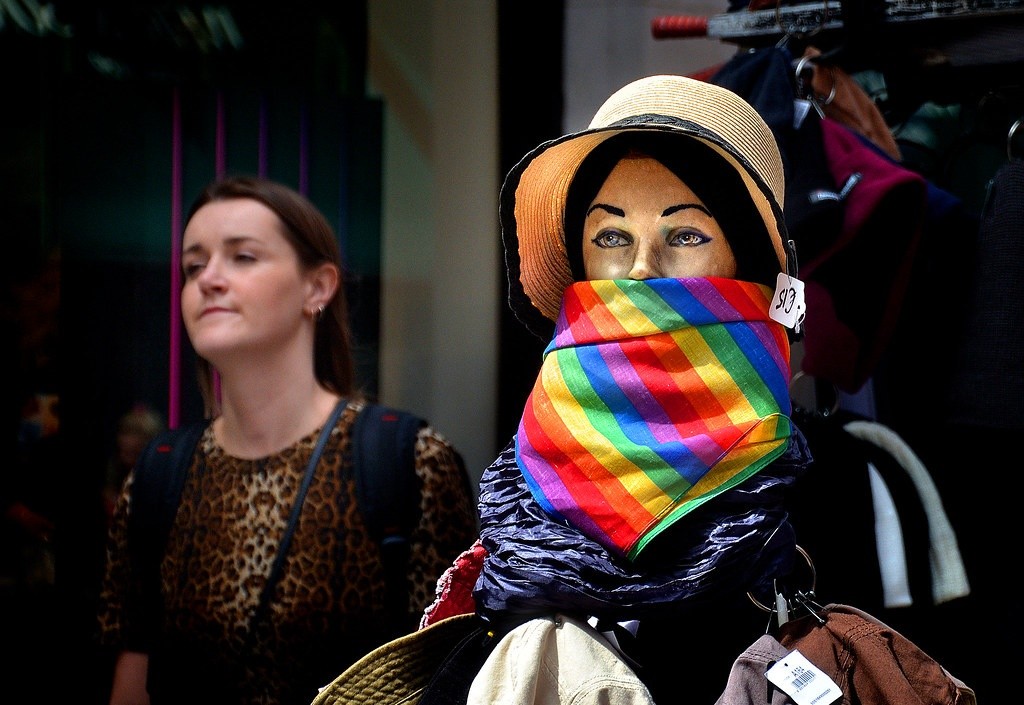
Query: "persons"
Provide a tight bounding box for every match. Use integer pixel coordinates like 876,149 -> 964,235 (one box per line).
0,392 -> 167,705
96,176 -> 482,705
414,74 -> 938,705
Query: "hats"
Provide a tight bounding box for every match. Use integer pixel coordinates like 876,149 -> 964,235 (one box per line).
312,613 -> 494,705
499,75 -> 792,343
467,613 -> 656,705
